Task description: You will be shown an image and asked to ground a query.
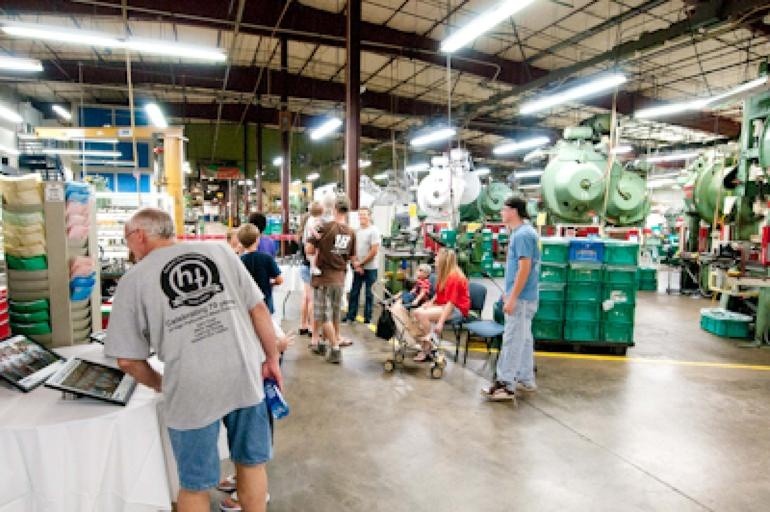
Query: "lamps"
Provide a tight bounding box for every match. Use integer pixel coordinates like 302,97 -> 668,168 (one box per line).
520,64 -> 628,115
438,0 -> 535,53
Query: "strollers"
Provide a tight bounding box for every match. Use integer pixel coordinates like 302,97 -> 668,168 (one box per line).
371,281 -> 447,380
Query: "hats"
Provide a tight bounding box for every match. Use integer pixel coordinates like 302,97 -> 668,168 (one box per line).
505,196 -> 531,220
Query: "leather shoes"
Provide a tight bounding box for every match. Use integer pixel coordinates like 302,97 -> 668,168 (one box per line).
298,327 -> 308,335
307,329 -> 321,338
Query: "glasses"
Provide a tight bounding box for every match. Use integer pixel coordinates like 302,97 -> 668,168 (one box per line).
121,230 -> 135,246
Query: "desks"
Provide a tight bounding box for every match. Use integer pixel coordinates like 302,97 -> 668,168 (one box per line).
0,344 -> 231,511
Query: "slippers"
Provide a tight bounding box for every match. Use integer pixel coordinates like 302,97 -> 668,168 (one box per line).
218,490 -> 270,512
218,475 -> 237,490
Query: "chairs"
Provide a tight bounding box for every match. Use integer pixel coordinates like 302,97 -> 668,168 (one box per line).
440,283 -> 487,363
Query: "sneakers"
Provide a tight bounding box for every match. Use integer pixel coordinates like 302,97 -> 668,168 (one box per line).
325,347 -> 342,363
480,386 -> 515,401
308,267 -> 321,276
517,382 -> 537,392
339,317 -> 356,326
307,342 -> 327,355
363,319 -> 372,326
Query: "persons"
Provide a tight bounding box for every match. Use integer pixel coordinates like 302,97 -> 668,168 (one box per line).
302,201 -> 325,277
302,199 -> 357,365
237,222 -> 284,315
296,212 -> 322,338
248,213 -> 275,260
395,263 -> 431,308
481,196 -> 541,401
341,207 -> 381,326
413,248 -> 470,360
104,207 -> 283,512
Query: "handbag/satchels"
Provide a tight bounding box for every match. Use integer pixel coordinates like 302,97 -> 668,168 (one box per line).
374,304 -> 395,341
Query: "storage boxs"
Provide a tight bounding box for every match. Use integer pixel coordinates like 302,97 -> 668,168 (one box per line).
701,311 -> 750,337
493,264 -> 505,277
443,226 -> 493,277
535,240 -> 643,342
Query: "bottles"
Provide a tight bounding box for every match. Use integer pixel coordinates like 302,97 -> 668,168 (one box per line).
264,378 -> 289,420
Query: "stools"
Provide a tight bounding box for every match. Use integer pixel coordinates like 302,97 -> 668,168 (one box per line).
463,319 -> 505,366
